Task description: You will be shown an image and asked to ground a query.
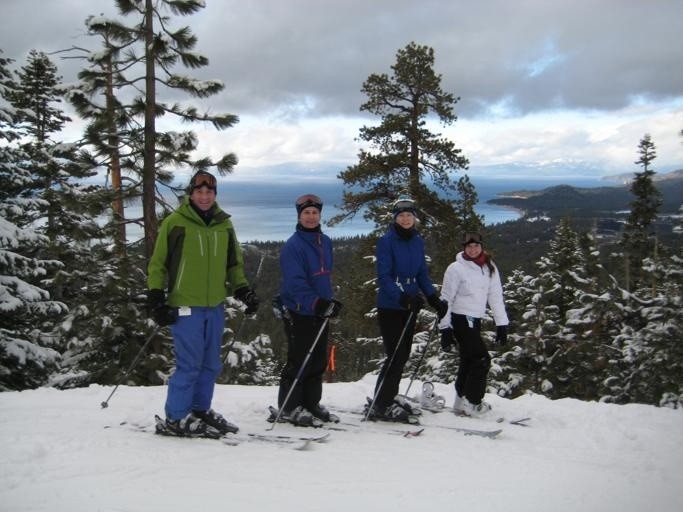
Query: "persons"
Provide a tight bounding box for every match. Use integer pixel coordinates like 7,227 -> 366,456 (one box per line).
146,171 -> 260,439
436,233 -> 510,418
372,196 -> 449,419
278,191 -> 343,427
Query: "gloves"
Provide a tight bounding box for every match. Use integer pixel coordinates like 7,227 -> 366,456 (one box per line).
233,287 -> 261,314
495,325 -> 508,347
312,296 -> 343,320
427,293 -> 449,320
145,288 -> 177,327
439,327 -> 458,353
398,292 -> 426,315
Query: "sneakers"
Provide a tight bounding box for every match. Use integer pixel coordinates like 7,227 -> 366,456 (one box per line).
279,402 -> 329,426
363,396 -> 415,422
165,408 -> 227,435
453,394 -> 505,423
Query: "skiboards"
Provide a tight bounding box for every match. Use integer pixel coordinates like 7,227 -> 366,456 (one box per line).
325,402 -> 504,438
104,395 -> 531,448
265,405 -> 424,438
106,419 -> 331,449
401,394 -> 530,425
